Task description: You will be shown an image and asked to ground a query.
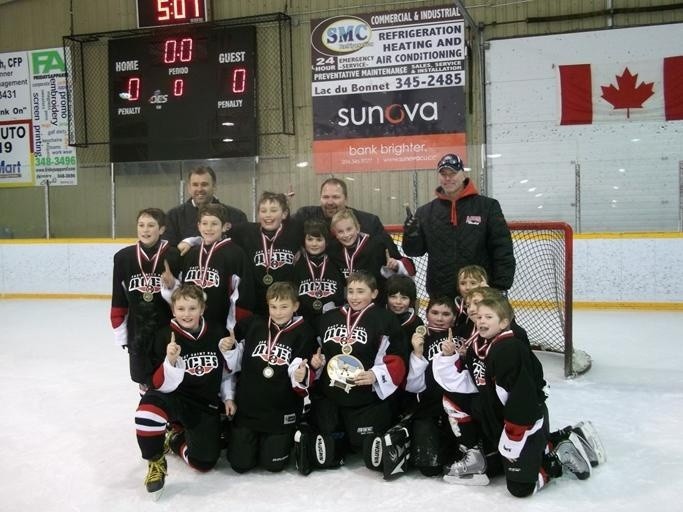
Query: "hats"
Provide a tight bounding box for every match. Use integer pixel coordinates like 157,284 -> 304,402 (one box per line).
438,154 -> 463,173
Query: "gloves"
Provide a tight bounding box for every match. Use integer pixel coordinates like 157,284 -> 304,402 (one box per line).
404,207 -> 420,236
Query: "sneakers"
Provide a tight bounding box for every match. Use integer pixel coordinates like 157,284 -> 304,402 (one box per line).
448,444 -> 486,475
565,425 -> 598,467
145,457 -> 167,493
550,440 -> 589,480
164,423 -> 178,454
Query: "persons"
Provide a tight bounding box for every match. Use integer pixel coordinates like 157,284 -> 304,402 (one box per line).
280,177 -> 401,281
133,282 -> 239,494
402,151 -> 515,299
225,270 -> 604,500
276,218 -> 346,322
177,190 -> 305,325
109,208 -> 183,393
154,200 -> 256,328
162,166 -> 246,236
327,209 -> 416,291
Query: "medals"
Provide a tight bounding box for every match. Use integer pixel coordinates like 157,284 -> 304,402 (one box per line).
142,292 -> 153,303
201,291 -> 206,302
